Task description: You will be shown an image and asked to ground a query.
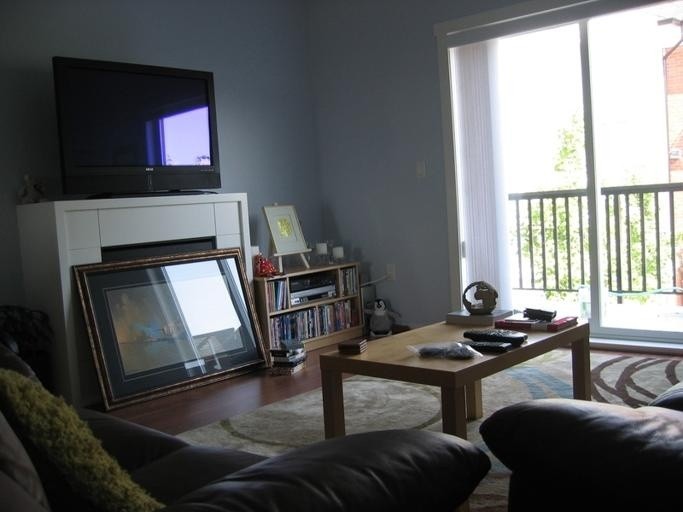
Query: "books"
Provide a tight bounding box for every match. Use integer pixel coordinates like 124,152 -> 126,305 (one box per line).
445,305 -> 511,327
260,267 -> 367,377
503,310 -> 540,326
494,312 -> 578,332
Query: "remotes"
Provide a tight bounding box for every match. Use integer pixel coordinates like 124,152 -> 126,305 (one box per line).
460,340 -> 513,355
464,329 -> 529,343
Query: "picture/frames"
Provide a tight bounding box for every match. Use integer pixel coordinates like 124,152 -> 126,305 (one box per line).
69,246 -> 272,412
261,204 -> 312,257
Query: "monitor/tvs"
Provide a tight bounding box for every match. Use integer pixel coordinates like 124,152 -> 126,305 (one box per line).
51,54 -> 221,199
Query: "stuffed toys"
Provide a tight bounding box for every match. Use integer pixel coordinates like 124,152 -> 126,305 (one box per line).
362,296 -> 402,336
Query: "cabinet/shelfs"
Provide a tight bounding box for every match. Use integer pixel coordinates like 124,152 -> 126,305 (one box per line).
252,261 -> 366,363
13,191 -> 259,411
